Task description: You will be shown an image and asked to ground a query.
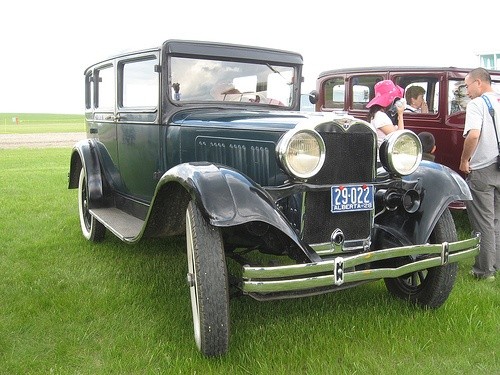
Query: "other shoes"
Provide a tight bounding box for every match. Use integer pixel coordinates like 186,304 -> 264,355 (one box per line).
468,270 -> 494,282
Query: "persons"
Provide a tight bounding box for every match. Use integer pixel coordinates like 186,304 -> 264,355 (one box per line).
459,67 -> 500,282
417,132 -> 436,162
404,85 -> 429,114
366,79 -> 406,169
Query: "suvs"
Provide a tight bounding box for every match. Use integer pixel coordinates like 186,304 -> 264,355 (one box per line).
67,40 -> 481,355
316,66 -> 500,210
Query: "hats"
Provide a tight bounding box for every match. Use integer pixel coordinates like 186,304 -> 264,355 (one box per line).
366,80 -> 404,108
